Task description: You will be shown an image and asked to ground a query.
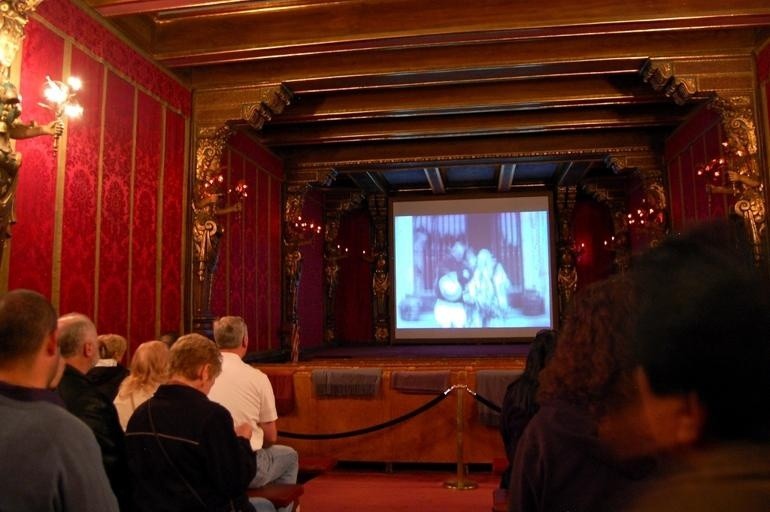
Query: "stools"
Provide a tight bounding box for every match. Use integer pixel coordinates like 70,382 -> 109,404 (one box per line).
248,484 -> 304,512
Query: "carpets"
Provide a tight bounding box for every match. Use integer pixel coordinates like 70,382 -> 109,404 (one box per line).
298,471 -> 502,512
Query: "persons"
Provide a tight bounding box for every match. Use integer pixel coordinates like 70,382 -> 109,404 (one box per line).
0,290 -> 120,512
125,333 -> 275,512
435,241 -> 470,327
501,330 -> 556,489
206,317 -> 300,512
509,275 -> 683,511
113,341 -> 171,432
49,311 -> 124,484
634,225 -> 770,511
87,334 -> 131,403
470,249 -> 509,328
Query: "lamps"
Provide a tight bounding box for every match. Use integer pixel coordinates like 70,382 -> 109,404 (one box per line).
204,176 -> 232,216
36,75 -> 83,157
721,142 -> 742,197
234,182 -> 247,224
697,158 -> 724,207
